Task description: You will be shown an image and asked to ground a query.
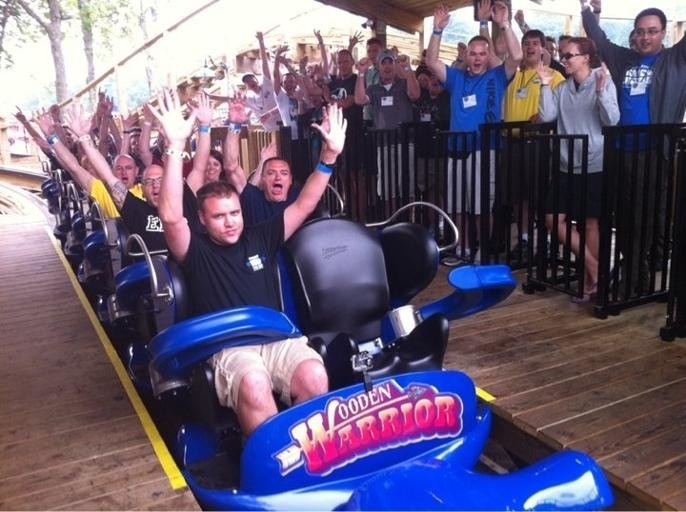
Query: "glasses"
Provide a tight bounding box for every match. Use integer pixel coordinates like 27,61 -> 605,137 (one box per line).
559,51 -> 586,60
633,26 -> 663,36
139,176 -> 164,187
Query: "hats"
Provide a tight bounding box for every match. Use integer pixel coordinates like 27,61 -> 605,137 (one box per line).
375,49 -> 398,65
240,71 -> 257,82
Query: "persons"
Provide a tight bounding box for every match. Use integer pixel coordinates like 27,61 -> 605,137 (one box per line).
13,1 -> 685,439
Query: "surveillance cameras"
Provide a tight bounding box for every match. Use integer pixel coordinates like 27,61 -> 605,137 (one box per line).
361,20 -> 373,28
